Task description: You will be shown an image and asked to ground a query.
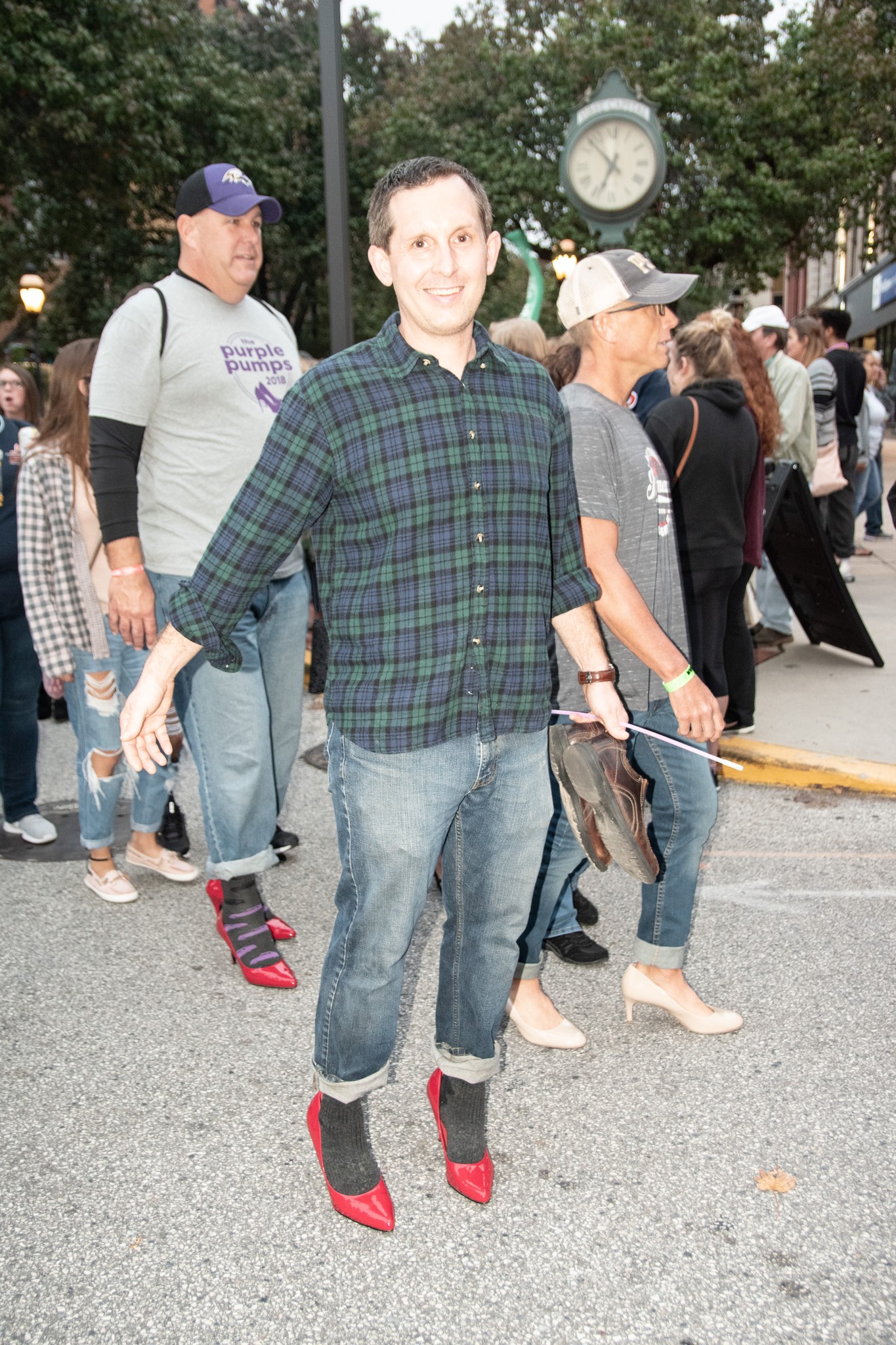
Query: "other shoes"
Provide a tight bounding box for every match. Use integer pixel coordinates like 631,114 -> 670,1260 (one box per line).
315,696 -> 324,710
83,864 -> 139,902
722,716 -> 755,734
748,620 -> 764,635
864,532 -> 893,541
837,558 -> 855,582
853,546 -> 873,556
750,627 -> 793,648
124,843 -> 199,882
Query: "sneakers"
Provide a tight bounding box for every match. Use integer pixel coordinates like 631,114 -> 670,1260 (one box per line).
2,813 -> 58,844
571,888 -> 599,926
562,731 -> 659,885
269,824 -> 299,854
547,720 -> 613,872
155,800 -> 190,858
541,931 -> 609,965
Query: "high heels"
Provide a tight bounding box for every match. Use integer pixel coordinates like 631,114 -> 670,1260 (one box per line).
206,879 -> 296,939
621,963 -> 744,1034
217,909 -> 297,988
427,1067 -> 494,1204
308,1090 -> 395,1232
504,998 -> 586,1049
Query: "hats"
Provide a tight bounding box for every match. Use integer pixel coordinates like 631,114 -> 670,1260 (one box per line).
556,249 -> 699,330
741,305 -> 790,333
176,164 -> 282,223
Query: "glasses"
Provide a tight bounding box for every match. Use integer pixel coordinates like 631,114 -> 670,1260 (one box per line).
587,302 -> 665,320
0,381 -> 24,388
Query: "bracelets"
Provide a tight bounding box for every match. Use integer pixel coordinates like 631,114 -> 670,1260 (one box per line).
659,666 -> 696,694
110,562 -> 146,579
577,663 -> 617,687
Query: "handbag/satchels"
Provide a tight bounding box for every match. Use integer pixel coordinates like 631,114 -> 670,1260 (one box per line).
809,442 -> 849,497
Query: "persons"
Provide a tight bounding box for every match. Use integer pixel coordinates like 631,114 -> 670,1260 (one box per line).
88,162 -> 317,989
125,134 -> 598,1225
501,245 -> 741,1046
0,278 -> 894,970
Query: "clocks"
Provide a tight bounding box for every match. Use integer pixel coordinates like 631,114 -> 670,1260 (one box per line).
558,67 -> 667,222
